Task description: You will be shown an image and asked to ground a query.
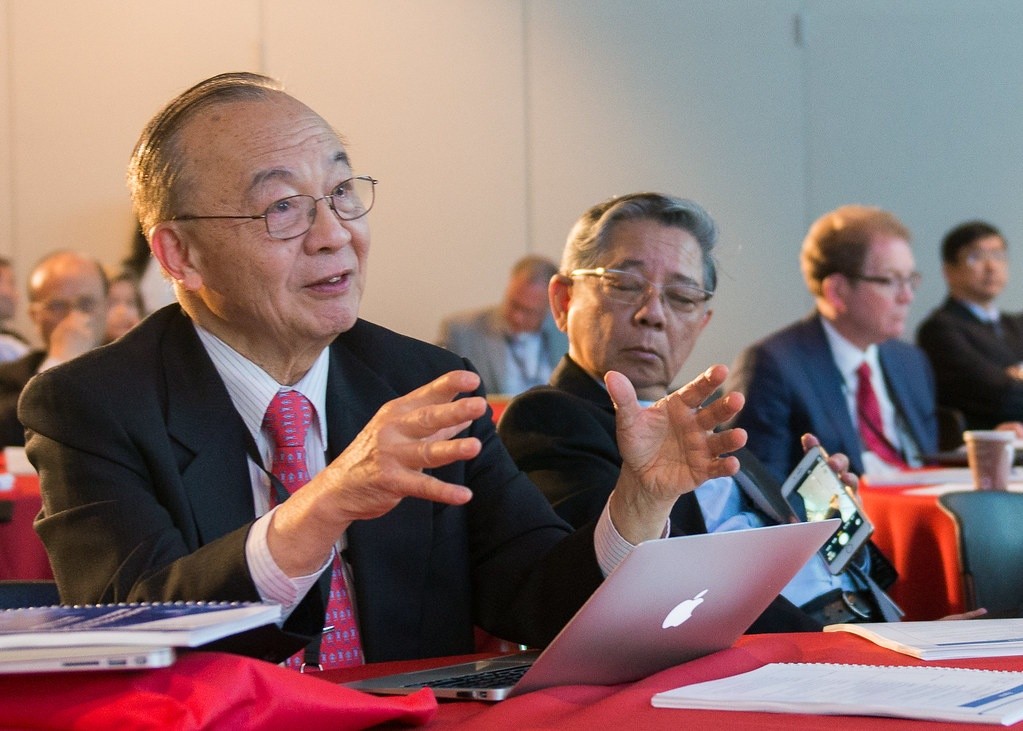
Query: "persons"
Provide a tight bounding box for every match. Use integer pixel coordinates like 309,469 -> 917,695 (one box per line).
0,221 -> 180,448
917,217 -> 1022,442
824,491 -> 844,524
724,202 -> 1023,512
429,255 -> 567,400
491,190 -> 906,639
18,71 -> 748,680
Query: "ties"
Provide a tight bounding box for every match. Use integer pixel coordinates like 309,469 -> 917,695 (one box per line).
855,365 -> 941,473
266,390 -> 361,671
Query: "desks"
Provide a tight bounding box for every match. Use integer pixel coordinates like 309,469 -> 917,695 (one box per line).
309,622 -> 1021,731
848,459 -> 1023,623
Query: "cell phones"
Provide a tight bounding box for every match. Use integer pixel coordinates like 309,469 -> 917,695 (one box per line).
781,446 -> 873,576
2,644 -> 176,673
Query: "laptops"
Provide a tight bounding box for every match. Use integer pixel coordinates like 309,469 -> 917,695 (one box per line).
333,519 -> 849,702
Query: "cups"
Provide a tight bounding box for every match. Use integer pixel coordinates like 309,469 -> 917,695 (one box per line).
963,429 -> 1016,491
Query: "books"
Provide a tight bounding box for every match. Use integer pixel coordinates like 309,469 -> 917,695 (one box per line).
822,618 -> 1023,661
650,661 -> 1023,728
0,601 -> 282,648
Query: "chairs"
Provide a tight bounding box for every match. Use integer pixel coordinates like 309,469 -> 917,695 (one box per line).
933,486 -> 1021,621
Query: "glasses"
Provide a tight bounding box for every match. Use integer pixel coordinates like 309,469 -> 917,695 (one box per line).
166,175 -> 380,241
846,270 -> 924,292
570,265 -> 714,312
